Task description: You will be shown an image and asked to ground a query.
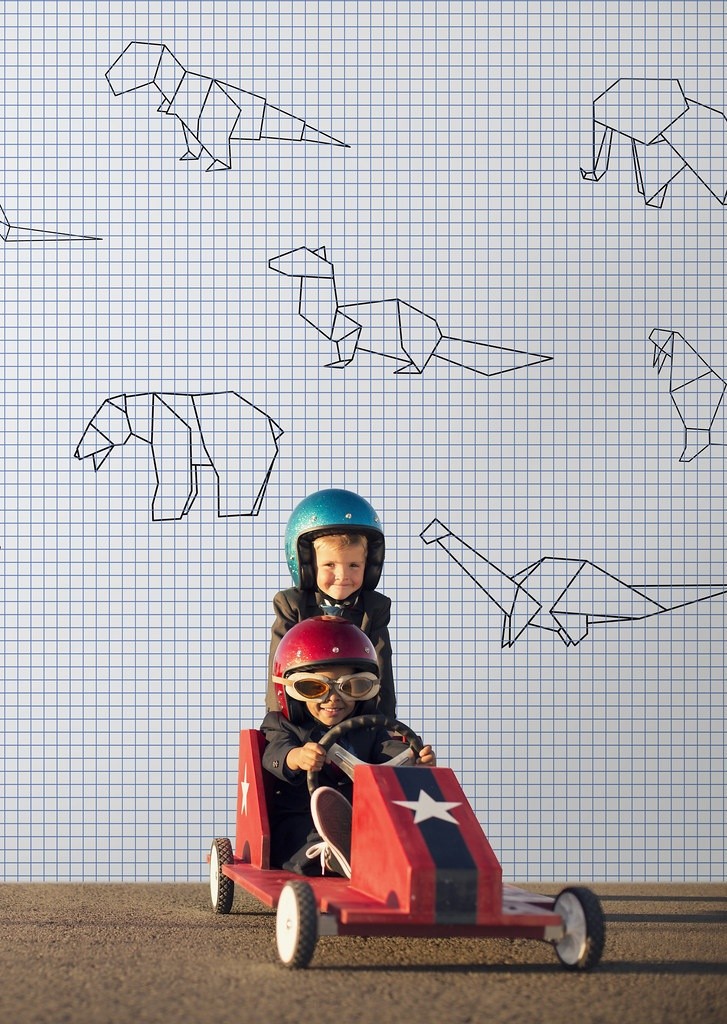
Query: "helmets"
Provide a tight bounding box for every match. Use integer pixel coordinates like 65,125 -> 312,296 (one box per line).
286,489 -> 386,600
273,614 -> 381,723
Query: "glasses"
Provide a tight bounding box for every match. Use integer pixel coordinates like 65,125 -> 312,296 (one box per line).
272,672 -> 381,703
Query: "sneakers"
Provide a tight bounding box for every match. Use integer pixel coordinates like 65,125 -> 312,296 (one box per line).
305,787 -> 354,882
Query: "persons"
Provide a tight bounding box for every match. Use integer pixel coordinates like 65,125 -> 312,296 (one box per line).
258,615 -> 435,881
264,489 -> 396,713
